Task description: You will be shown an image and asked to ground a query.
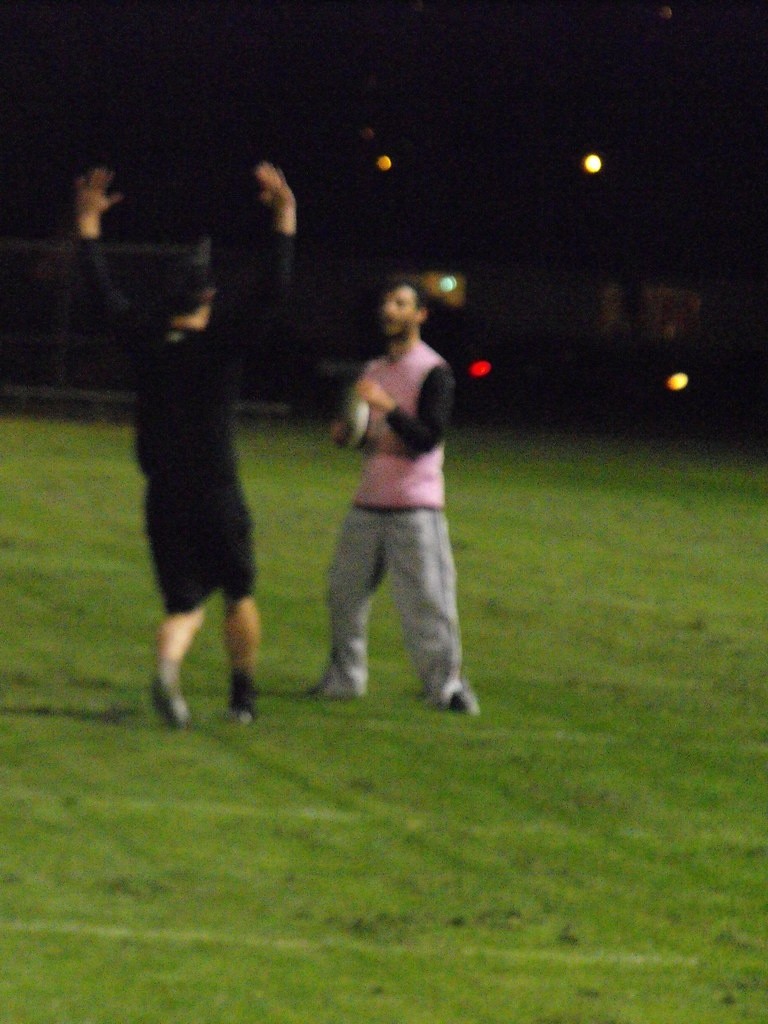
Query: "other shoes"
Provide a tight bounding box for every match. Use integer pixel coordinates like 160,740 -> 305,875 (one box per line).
449,685 -> 479,716
305,682 -> 354,700
150,670 -> 190,727
229,679 -> 254,724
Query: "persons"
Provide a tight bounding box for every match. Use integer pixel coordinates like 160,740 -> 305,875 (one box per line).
74,160 -> 299,728
301,278 -> 484,717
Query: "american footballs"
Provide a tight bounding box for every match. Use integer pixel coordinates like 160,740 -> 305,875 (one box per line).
340,384 -> 371,449
